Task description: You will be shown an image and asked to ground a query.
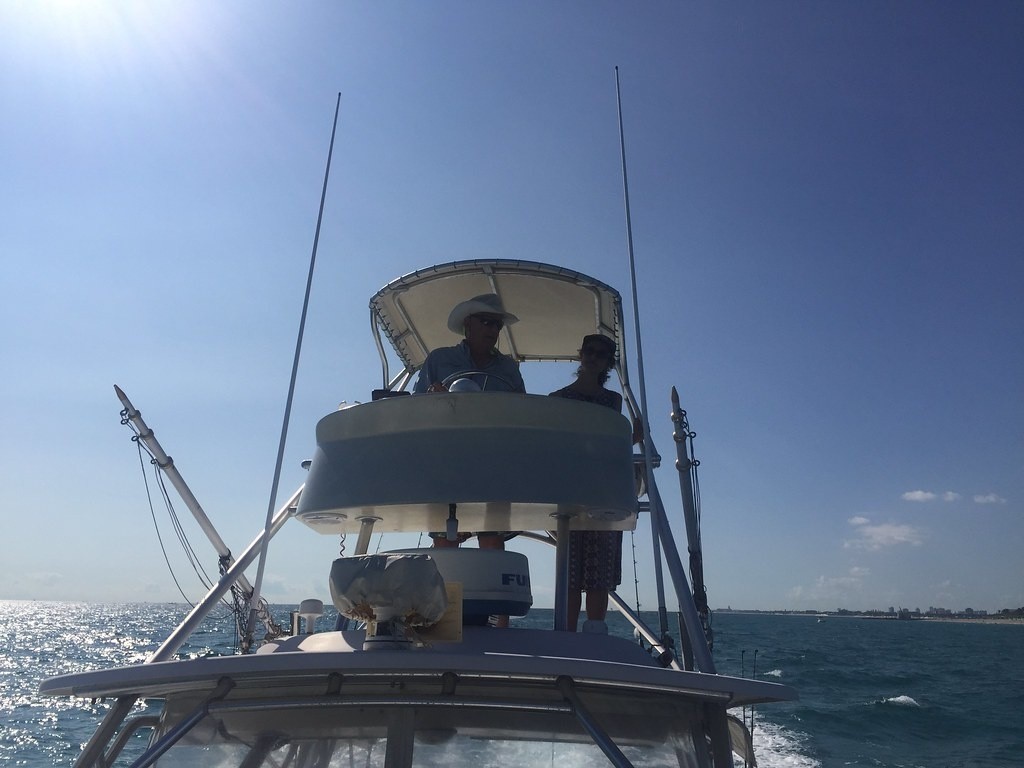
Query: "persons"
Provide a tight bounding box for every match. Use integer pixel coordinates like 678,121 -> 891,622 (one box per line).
412,294 -> 527,638
548,332 -> 640,639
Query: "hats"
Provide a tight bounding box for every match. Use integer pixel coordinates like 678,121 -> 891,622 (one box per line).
447,293 -> 520,335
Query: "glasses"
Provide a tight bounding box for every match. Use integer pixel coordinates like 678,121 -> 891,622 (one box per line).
471,314 -> 503,331
582,346 -> 609,360
